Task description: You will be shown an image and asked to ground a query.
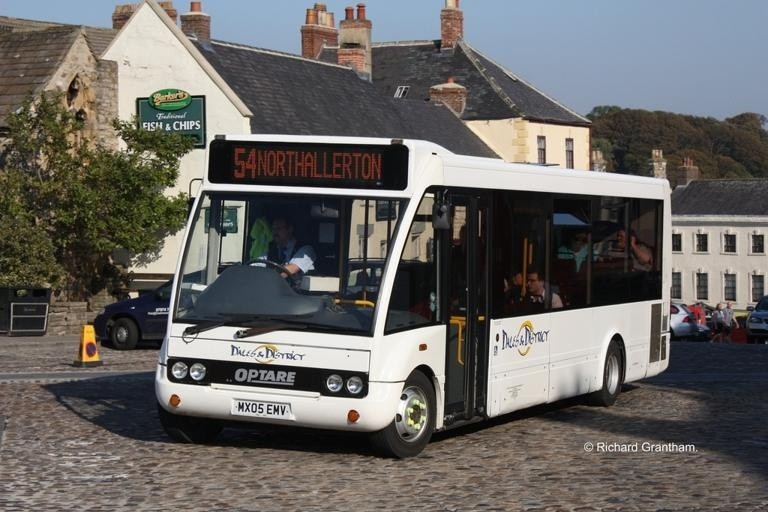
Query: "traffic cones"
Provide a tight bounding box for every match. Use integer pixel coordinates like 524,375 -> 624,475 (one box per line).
70,323 -> 104,367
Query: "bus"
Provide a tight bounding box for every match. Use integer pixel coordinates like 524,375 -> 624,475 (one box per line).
150,134 -> 675,461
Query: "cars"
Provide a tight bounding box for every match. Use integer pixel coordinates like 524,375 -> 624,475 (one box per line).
95,263 -> 239,352
670,295 -> 767,345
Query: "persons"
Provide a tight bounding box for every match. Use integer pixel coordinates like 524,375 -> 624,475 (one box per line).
709,302 -> 739,343
503,268 -> 563,308
602,227 -> 653,275
266,216 -> 317,285
558,233 -> 599,271
